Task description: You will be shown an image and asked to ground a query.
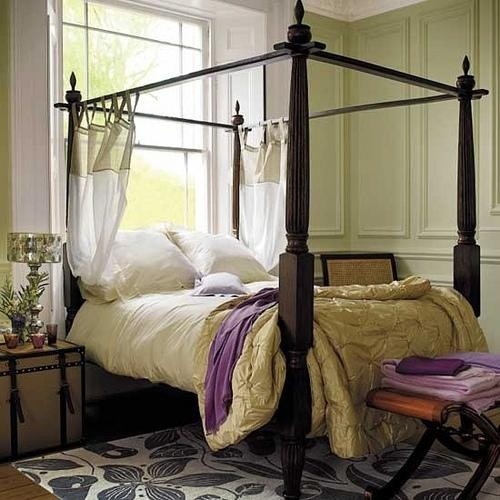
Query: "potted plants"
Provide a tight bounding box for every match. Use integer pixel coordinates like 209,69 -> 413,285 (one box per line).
0,265 -> 53,346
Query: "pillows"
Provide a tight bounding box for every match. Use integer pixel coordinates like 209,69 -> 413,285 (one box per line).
112,218 -> 202,304
191,271 -> 253,298
163,220 -> 279,284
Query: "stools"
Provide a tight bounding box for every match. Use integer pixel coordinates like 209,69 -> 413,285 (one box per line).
363,385 -> 500,499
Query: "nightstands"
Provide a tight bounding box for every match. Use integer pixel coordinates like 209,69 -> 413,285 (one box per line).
0,337 -> 89,465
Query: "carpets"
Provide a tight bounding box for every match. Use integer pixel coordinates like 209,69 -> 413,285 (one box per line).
6,420 -> 500,500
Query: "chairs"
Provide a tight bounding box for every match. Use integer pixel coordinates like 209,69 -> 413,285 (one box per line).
319,250 -> 399,288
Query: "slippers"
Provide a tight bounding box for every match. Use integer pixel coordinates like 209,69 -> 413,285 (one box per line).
246,428 -> 274,455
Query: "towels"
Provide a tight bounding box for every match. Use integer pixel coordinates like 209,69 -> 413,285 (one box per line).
396,353 -> 472,377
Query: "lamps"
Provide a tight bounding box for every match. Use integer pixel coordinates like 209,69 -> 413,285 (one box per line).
6,230 -> 63,343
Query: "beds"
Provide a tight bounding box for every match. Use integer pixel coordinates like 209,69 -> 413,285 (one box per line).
52,0 -> 490,500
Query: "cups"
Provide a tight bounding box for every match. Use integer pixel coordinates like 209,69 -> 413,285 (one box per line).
5,333 -> 22,349
29,333 -> 45,349
46,322 -> 58,344
